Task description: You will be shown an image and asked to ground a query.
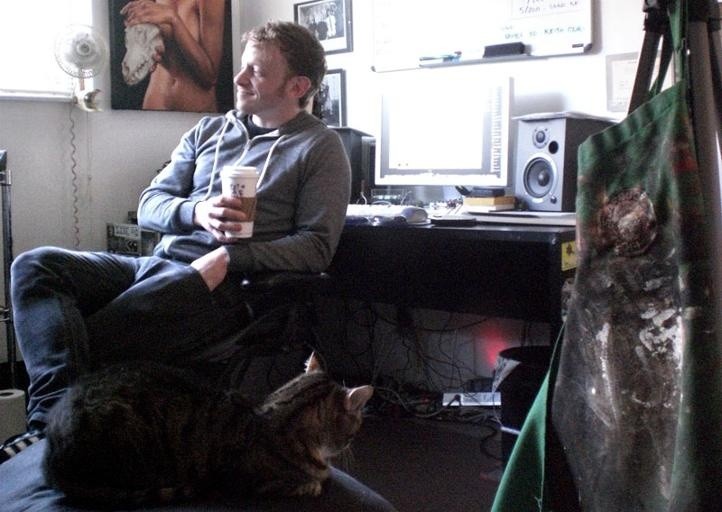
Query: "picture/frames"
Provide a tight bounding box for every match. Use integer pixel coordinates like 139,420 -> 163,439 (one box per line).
293,1 -> 353,129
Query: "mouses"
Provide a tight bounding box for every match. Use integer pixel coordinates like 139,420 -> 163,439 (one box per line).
400,207 -> 428,223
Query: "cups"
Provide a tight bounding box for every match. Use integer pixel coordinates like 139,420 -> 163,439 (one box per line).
216,166 -> 263,236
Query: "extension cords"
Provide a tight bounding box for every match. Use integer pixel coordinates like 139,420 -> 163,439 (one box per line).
442,392 -> 501,406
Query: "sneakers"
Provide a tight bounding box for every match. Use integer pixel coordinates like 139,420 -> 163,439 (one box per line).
0,426 -> 48,465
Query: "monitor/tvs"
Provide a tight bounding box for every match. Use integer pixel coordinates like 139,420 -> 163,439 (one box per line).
374,76 -> 512,197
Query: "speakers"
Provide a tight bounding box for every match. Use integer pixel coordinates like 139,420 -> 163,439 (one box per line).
515,117 -> 613,212
334,128 -> 388,206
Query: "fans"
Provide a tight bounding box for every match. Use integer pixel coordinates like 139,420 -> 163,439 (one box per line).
52,23 -> 108,114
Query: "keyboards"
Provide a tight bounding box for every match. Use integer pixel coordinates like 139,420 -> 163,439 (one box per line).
347,204 -> 409,218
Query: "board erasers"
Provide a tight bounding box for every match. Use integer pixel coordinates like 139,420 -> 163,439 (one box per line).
482,42 -> 524,58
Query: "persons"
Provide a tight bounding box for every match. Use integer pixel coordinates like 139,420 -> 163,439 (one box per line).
303,4 -> 344,41
311,82 -> 339,127
119,0 -> 227,114
0,21 -> 354,460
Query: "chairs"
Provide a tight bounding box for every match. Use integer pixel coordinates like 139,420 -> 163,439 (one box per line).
171,216 -> 371,396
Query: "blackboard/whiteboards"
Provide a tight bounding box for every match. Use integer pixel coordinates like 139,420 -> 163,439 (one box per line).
370,0 -> 595,74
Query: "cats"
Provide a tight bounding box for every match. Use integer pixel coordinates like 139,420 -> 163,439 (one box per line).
39,350 -> 374,512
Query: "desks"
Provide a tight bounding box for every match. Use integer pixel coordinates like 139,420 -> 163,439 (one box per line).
320,222 -> 577,366
